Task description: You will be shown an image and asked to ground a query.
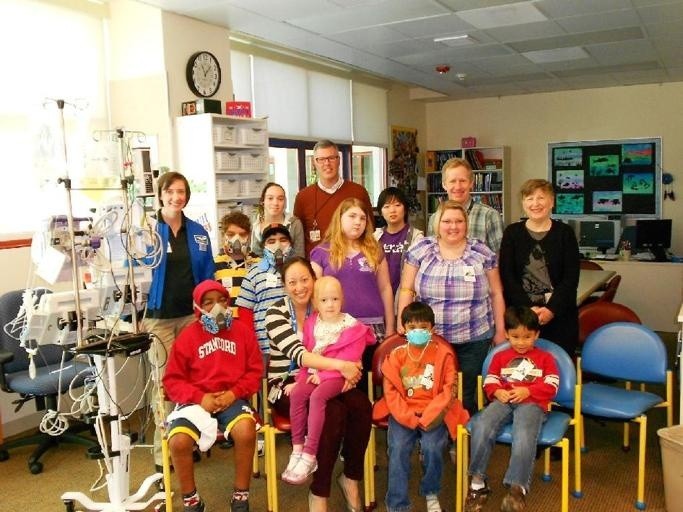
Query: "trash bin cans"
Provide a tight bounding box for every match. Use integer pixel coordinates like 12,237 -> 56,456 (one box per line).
657,424 -> 683,512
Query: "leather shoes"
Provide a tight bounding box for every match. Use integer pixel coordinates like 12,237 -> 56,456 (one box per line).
280,450 -> 321,486
335,471 -> 365,512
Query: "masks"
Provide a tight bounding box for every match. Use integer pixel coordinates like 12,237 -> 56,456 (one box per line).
406,329 -> 433,347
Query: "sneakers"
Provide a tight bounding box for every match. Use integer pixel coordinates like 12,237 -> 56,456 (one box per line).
425,494 -> 443,512
499,492 -> 529,512
306,487 -> 330,512
182,499 -> 208,512
229,497 -> 250,512
463,479 -> 492,512
153,436 -> 271,474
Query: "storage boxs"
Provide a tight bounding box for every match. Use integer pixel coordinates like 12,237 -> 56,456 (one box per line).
213,124 -> 267,249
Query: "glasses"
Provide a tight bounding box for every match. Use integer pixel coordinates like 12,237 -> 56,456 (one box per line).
314,155 -> 340,164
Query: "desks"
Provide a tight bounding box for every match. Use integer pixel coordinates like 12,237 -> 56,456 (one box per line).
576,270 -> 617,307
588,260 -> 683,333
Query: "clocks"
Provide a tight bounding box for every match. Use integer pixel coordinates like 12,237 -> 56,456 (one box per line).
186,50 -> 221,97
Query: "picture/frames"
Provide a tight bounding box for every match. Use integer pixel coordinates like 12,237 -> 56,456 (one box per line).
389,126 -> 418,160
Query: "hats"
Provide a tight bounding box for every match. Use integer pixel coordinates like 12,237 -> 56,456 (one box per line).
261,222 -> 293,244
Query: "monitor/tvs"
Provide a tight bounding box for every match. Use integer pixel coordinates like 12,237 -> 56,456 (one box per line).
547,137 -> 662,220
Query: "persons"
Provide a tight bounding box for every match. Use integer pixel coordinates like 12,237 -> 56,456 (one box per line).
124,140 -> 580,511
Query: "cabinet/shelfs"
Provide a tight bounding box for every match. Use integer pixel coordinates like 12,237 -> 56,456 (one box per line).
175,114 -> 271,258
426,146 -> 512,237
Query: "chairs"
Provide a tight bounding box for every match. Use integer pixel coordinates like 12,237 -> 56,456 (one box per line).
368,332 -> 463,512
0,288 -> 111,474
576,302 -> 646,452
158,373 -> 273,512
543,322 -> 673,510
463,338 -> 582,512
263,354 -> 370,511
580,261 -> 603,270
582,276 -> 621,301
